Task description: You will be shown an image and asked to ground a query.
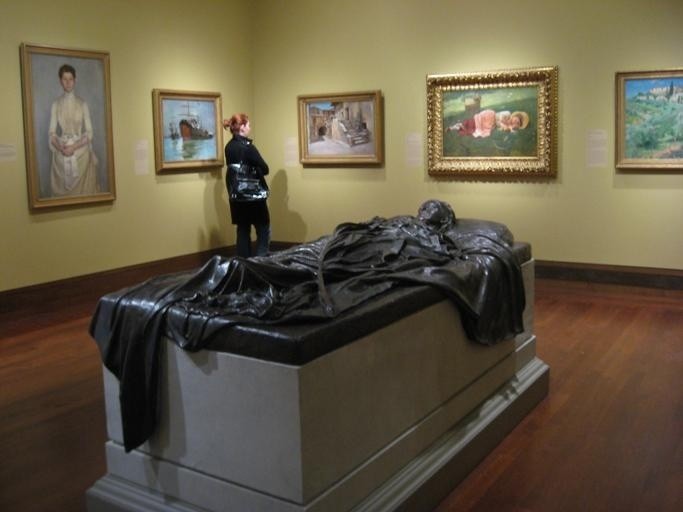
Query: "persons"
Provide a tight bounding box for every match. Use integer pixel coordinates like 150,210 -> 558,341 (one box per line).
205,199 -> 455,295
445,110 -> 529,138
49,64 -> 98,196
224,114 -> 273,256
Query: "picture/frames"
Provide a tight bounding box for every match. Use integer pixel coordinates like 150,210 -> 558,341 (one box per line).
20,43 -> 116,209
297,90 -> 383,163
615,68 -> 683,170
151,89 -> 224,173
426,66 -> 558,177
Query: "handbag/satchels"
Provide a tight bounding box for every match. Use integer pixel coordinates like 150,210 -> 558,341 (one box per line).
228,176 -> 270,204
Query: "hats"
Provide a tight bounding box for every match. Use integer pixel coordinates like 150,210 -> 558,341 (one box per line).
512,111 -> 529,129
